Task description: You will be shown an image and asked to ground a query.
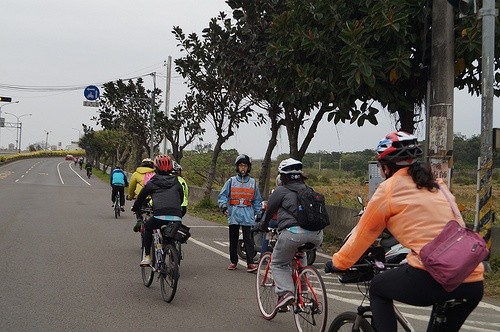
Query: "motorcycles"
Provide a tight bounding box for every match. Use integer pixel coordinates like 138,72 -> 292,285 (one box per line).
338,196 -> 411,272
236,188 -> 322,266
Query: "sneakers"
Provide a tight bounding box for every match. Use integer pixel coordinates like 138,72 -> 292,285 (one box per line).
140,255 -> 151,267
274,291 -> 294,310
228,263 -> 238,269
301,285 -> 308,293
247,264 -> 257,272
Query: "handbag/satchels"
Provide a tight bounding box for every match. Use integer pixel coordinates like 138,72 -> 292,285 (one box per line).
419,220 -> 489,292
338,246 -> 385,283
165,221 -> 191,244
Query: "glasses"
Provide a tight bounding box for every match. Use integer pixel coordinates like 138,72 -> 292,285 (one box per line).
238,164 -> 248,168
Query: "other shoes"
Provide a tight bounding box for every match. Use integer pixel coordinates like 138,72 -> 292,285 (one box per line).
111,203 -> 115,207
133,219 -> 142,232
121,206 -> 125,212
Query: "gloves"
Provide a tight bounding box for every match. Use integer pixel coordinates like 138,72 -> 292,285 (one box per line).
326,260 -> 340,274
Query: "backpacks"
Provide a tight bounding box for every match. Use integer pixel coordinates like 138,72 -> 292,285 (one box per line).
282,185 -> 331,231
138,172 -> 155,189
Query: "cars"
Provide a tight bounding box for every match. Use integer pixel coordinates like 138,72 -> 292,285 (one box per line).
66,154 -> 74,160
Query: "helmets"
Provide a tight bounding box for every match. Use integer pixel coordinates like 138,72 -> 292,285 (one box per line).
142,158 -> 154,168
154,154 -> 173,174
277,157 -> 303,179
375,131 -> 423,163
172,160 -> 183,173
235,154 -> 252,165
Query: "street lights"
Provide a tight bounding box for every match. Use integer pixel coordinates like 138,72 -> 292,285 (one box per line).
4,112 -> 33,155
72,127 -> 81,139
0,100 -> 20,123
44,129 -> 54,151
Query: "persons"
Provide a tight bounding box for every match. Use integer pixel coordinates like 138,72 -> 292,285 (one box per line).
255,158 -> 324,311
131,153 -> 184,264
326,131 -> 485,332
74,156 -> 85,164
217,154 -> 263,272
85,161 -> 92,176
79,159 -> 83,170
110,167 -> 128,213
169,161 -> 189,217
126,158 -> 156,232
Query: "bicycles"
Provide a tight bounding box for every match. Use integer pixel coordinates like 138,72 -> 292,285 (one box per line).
324,263 -> 414,332
255,222 -> 328,332
110,184 -> 128,218
129,197 -> 184,303
